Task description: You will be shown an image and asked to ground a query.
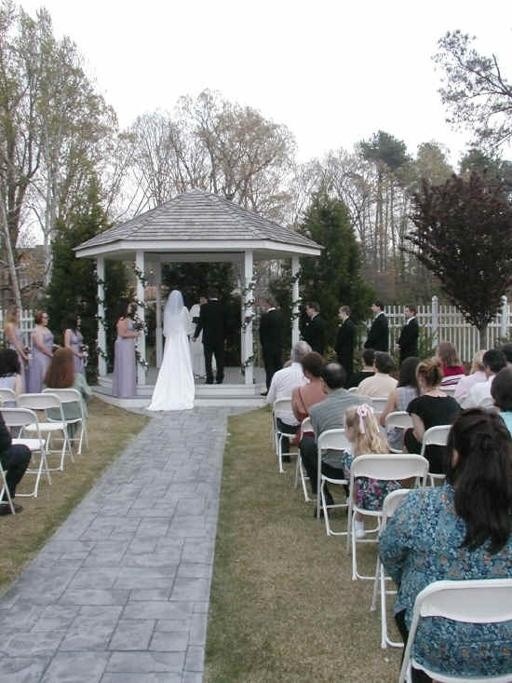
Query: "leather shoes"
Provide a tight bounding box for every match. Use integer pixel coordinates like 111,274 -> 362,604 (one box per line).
313,494 -> 333,518
0,502 -> 23,516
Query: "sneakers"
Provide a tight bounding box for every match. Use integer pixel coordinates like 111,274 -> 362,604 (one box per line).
351,517 -> 365,538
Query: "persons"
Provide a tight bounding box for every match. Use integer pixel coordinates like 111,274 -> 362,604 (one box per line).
455,350 -> 487,405
27,310 -> 58,411
63,314 -> 88,377
264,341 -> 315,463
0,350 -> 22,408
41,348 -> 91,450
334,306 -> 356,374
192,286 -> 225,383
257,295 -> 287,396
146,290 -> 197,411
379,357 -> 420,450
188,304 -> 217,378
301,301 -> 328,356
2,305 -> 29,393
0,410 -> 32,517
433,339 -> 465,387
364,300 -> 389,352
199,295 -> 208,307
291,355 -> 333,446
350,350 -> 377,387
490,367 -> 511,436
394,304 -> 419,368
110,300 -> 142,399
354,351 -> 398,398
376,408 -> 512,683
298,363 -> 371,519
341,404 -> 403,538
402,357 -> 465,485
463,350 -> 507,410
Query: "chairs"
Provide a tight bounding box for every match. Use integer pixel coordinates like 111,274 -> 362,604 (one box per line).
0,408 -> 53,499
348,387 -> 358,393
384,411 -> 413,455
346,455 -> 428,582
370,487 -> 409,649
15,392 -> 76,471
0,458 -> 16,515
42,388 -> 89,454
270,397 -> 299,474
294,417 -> 316,502
315,428 -> 381,537
398,580 -> 512,683
414,424 -> 452,486
439,388 -> 455,396
370,398 -> 389,413
1,388 -> 16,408
